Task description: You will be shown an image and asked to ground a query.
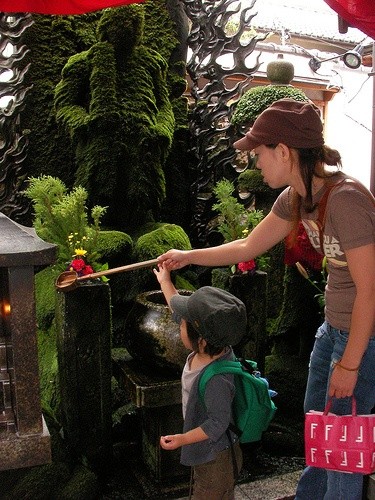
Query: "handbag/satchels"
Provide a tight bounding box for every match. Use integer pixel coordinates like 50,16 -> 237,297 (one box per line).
304,393 -> 375,474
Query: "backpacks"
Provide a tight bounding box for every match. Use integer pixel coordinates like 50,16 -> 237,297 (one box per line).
200,359 -> 276,443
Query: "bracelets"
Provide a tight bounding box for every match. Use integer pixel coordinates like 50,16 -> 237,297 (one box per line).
334,357 -> 363,375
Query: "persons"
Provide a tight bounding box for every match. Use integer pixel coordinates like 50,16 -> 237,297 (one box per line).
152,258 -> 279,499
155,99 -> 375,500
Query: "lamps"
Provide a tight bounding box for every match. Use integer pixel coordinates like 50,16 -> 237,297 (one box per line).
343,44 -> 364,69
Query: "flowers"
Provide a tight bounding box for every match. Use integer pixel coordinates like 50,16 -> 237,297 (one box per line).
231,229 -> 256,274
52,232 -> 110,283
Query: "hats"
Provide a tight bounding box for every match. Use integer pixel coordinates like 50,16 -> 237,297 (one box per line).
170,286 -> 247,347
233,98 -> 325,150
